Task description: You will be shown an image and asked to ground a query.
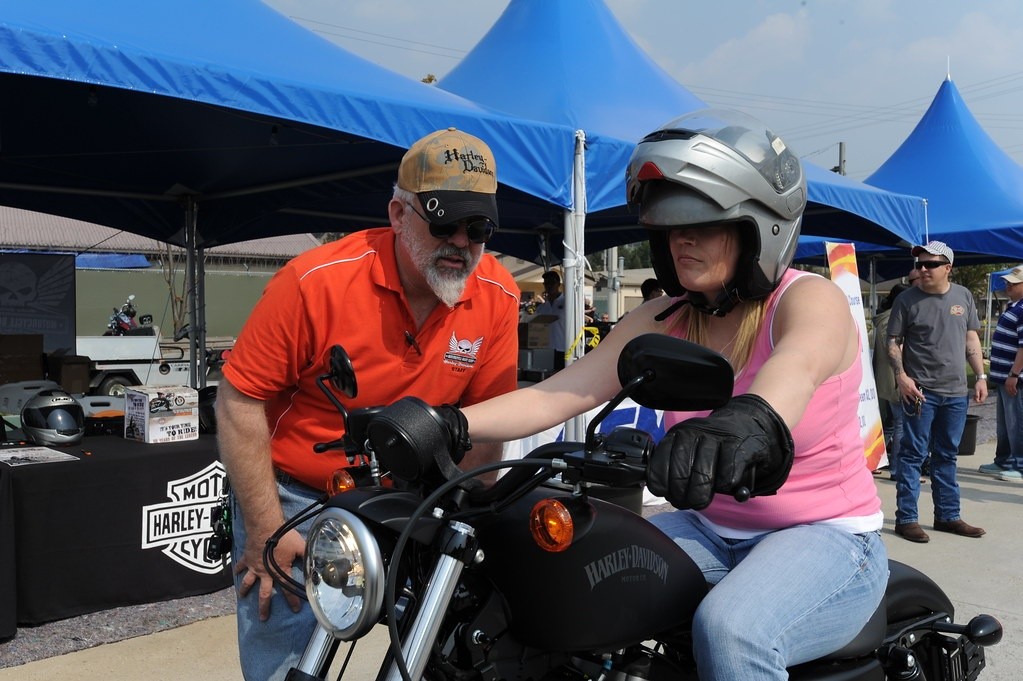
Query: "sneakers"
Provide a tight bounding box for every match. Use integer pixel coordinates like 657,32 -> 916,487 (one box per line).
979,462 -> 1007,473
998,469 -> 1023,482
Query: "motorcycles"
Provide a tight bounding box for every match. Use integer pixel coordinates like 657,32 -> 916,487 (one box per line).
261,331 -> 1002,681
103,295 -> 160,336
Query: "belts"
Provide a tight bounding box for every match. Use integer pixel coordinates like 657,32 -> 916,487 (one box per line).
290,475 -> 326,493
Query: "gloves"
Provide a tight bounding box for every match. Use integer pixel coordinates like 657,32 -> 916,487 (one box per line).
645,393 -> 794,512
431,404 -> 473,465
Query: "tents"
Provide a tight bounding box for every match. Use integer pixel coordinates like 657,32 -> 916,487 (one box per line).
0,0 -> 587,443
790,76 -> 1023,355
434,0 -> 932,443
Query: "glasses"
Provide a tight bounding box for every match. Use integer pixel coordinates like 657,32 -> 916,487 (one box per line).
1004,280 -> 1021,286
658,290 -> 663,294
543,281 -> 559,285
602,317 -> 608,319
914,261 -> 950,268
909,277 -> 920,285
405,201 -> 494,245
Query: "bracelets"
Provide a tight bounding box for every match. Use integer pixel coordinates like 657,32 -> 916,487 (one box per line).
976,374 -> 987,381
1009,371 -> 1019,377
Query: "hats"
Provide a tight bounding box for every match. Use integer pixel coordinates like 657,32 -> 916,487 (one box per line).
999,265 -> 1023,283
912,240 -> 954,267
398,127 -> 499,227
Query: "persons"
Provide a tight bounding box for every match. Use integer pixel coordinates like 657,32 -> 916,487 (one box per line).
978,267 -> 1023,481
585,295 -> 616,332
640,278 -> 664,306
368,109 -> 889,681
873,241 -> 989,542
214,127 -> 521,681
525,271 -> 566,372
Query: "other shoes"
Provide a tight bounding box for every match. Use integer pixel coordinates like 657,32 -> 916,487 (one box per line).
872,469 -> 882,474
890,475 -> 926,483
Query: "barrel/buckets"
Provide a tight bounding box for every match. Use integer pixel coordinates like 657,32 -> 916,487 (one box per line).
957,414 -> 983,456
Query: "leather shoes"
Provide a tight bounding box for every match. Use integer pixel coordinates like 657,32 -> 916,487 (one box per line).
895,523 -> 930,541
933,519 -> 986,536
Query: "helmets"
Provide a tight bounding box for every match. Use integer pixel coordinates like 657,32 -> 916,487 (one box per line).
624,108 -> 806,301
20,389 -> 87,446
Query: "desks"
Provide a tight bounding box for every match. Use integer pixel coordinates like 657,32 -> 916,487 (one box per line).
0,380 -> 682,641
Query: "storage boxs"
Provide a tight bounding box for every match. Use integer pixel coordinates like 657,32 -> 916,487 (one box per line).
124,384 -> 199,444
518,314 -> 560,349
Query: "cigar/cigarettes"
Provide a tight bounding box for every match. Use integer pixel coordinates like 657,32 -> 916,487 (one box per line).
914,386 -> 923,417
